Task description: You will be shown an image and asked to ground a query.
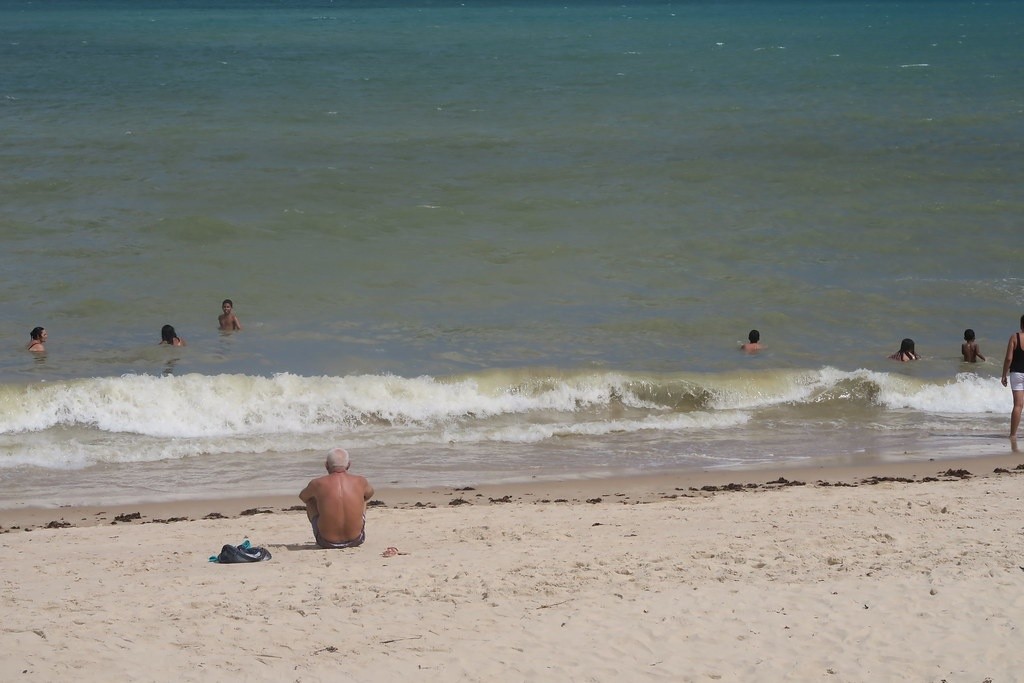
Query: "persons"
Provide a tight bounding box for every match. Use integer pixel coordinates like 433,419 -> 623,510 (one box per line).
27,326 -> 47,351
298,448 -> 375,547
888,337 -> 921,362
740,329 -> 767,353
158,324 -> 185,346
1002,314 -> 1024,452
961,328 -> 987,363
217,299 -> 242,331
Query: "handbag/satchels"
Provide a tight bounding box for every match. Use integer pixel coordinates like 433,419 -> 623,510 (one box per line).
209,539 -> 272,564
